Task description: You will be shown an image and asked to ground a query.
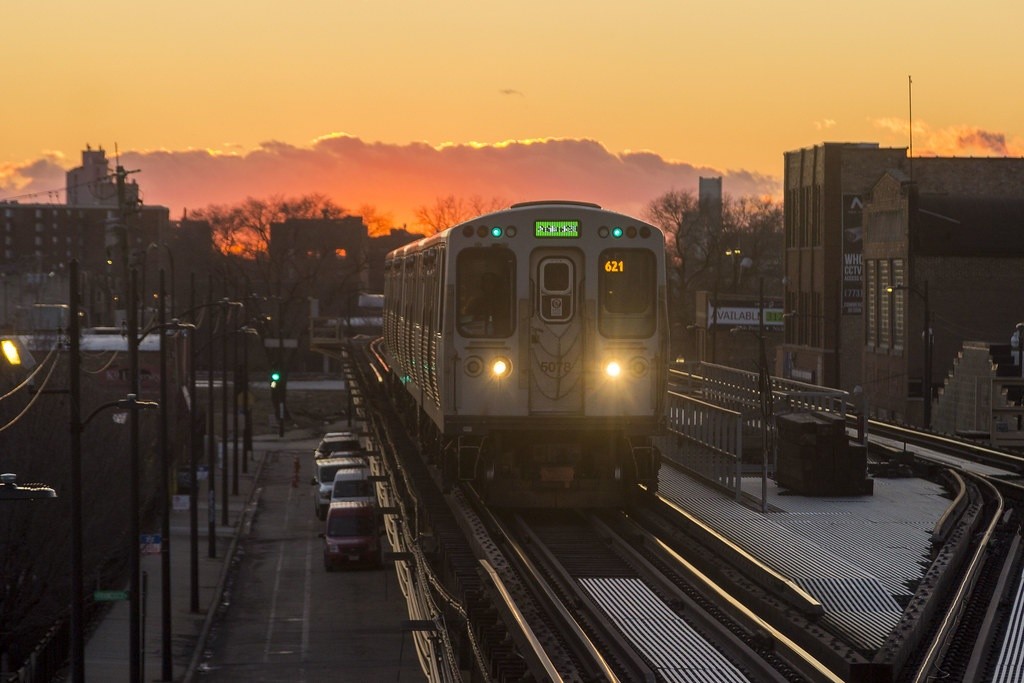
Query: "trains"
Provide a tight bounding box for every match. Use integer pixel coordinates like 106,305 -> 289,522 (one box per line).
381,199 -> 669,519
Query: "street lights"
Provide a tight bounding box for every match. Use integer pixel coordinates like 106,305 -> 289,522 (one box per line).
729,277 -> 769,506
157,294 -> 248,683
782,291 -> 842,388
69,256 -> 162,683
687,285 -> 719,365
129,268 -> 197,683
884,279 -> 933,431
189,324 -> 262,612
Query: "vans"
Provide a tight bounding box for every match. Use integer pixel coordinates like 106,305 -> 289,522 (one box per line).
313,432 -> 378,506
318,501 -> 385,573
